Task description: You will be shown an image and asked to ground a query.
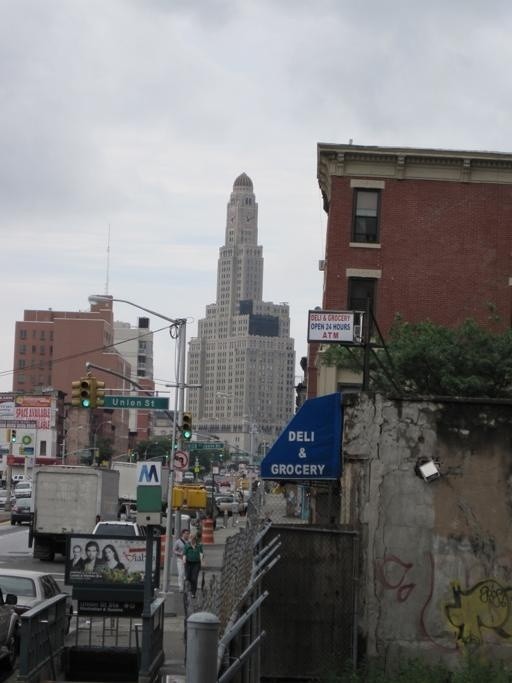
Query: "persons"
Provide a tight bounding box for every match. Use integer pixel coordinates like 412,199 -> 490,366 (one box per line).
71,544 -> 85,571
182,532 -> 205,598
78,540 -> 105,572
172,529 -> 192,593
102,544 -> 126,570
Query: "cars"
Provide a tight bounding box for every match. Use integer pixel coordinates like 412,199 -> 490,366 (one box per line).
0,565 -> 74,638
0,591 -> 19,679
90,518 -> 146,535
121,464 -> 265,527
0,473 -> 34,525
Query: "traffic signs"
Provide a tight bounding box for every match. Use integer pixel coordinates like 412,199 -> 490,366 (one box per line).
181,439 -> 225,448
96,392 -> 170,410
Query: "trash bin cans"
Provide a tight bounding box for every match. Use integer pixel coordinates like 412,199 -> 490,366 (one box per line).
180,514 -> 191,533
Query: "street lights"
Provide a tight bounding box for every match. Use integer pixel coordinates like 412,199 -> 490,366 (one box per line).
215,388 -> 252,495
91,419 -> 113,464
144,440 -> 158,459
60,424 -> 84,464
86,293 -> 192,593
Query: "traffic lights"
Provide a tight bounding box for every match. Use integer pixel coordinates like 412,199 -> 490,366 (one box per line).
182,409 -> 192,439
11,428 -> 16,441
79,375 -> 93,409
169,486 -> 184,508
92,376 -> 106,409
131,448 -> 134,456
70,379 -> 80,407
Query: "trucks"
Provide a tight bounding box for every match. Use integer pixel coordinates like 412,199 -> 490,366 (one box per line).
109,458 -> 170,522
26,463 -> 120,562
